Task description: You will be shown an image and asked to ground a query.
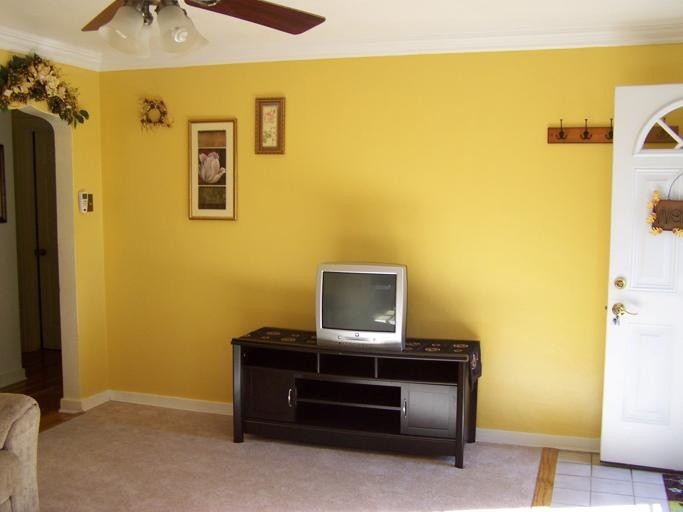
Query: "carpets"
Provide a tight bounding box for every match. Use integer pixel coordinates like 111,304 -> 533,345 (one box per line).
36,398 -> 546,511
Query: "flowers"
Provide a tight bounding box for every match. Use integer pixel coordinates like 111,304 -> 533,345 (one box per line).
0,46 -> 89,131
132,92 -> 173,135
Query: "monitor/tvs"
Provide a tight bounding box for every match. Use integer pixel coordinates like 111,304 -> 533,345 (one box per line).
314,264 -> 408,352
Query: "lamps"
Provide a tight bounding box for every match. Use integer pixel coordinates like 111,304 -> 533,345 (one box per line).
96,0 -> 211,59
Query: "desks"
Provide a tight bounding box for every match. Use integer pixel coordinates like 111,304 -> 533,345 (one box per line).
228,324 -> 483,470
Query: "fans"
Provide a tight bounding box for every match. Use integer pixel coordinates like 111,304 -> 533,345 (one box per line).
77,1 -> 326,36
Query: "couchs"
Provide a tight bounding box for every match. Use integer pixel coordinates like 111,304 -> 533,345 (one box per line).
0,390 -> 41,511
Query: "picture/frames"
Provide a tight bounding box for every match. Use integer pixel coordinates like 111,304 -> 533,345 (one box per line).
185,117 -> 239,222
253,96 -> 286,156
